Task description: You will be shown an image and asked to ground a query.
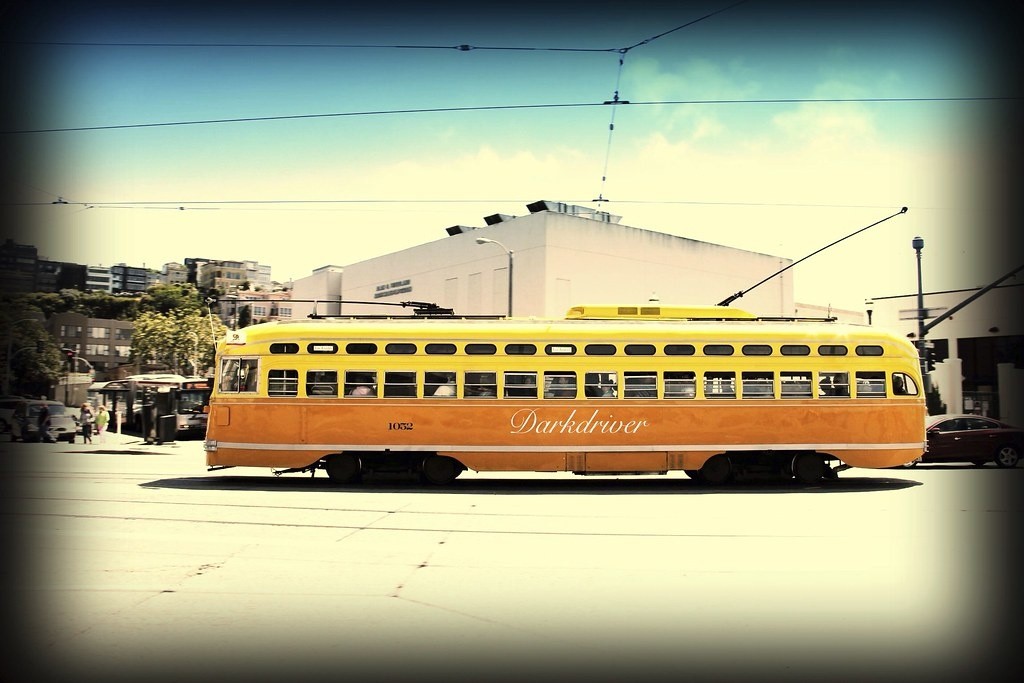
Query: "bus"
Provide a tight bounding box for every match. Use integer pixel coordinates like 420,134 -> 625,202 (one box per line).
202,304 -> 929,488
120,374 -> 214,433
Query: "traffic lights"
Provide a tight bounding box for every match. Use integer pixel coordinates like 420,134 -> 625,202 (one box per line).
66,348 -> 73,364
36,339 -> 45,355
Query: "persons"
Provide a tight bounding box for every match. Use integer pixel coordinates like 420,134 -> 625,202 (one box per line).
95,405 -> 110,444
228,363 -> 913,396
37,399 -> 58,443
79,402 -> 95,444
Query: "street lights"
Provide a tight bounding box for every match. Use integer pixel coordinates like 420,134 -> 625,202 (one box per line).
912,235 -> 928,389
477,236 -> 514,318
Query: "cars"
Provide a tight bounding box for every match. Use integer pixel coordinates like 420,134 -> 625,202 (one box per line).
896,414 -> 1024,469
0,397 -> 103,444
